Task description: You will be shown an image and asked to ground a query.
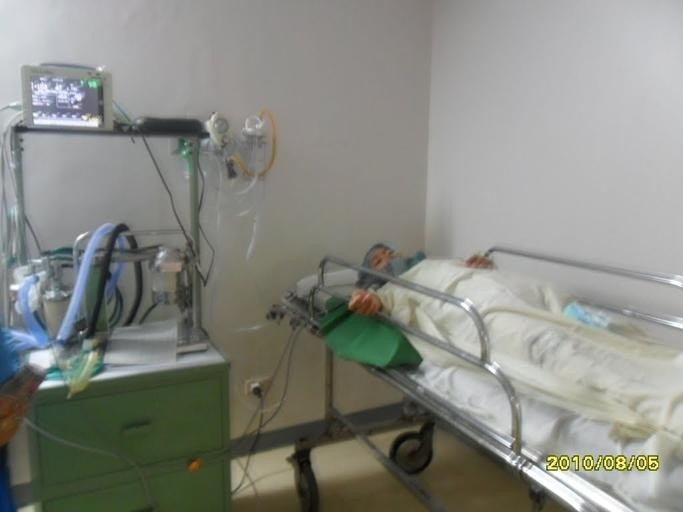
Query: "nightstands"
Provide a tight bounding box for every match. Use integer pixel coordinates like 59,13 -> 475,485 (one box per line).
22,316 -> 231,511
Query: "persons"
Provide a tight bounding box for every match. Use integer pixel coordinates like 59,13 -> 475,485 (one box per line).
346,241 -> 682,426
0,325 -> 48,512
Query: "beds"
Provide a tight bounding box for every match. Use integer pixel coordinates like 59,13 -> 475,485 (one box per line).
263,242 -> 683,511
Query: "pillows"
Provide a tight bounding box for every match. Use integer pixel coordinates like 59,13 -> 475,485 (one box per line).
296,266 -> 360,310
